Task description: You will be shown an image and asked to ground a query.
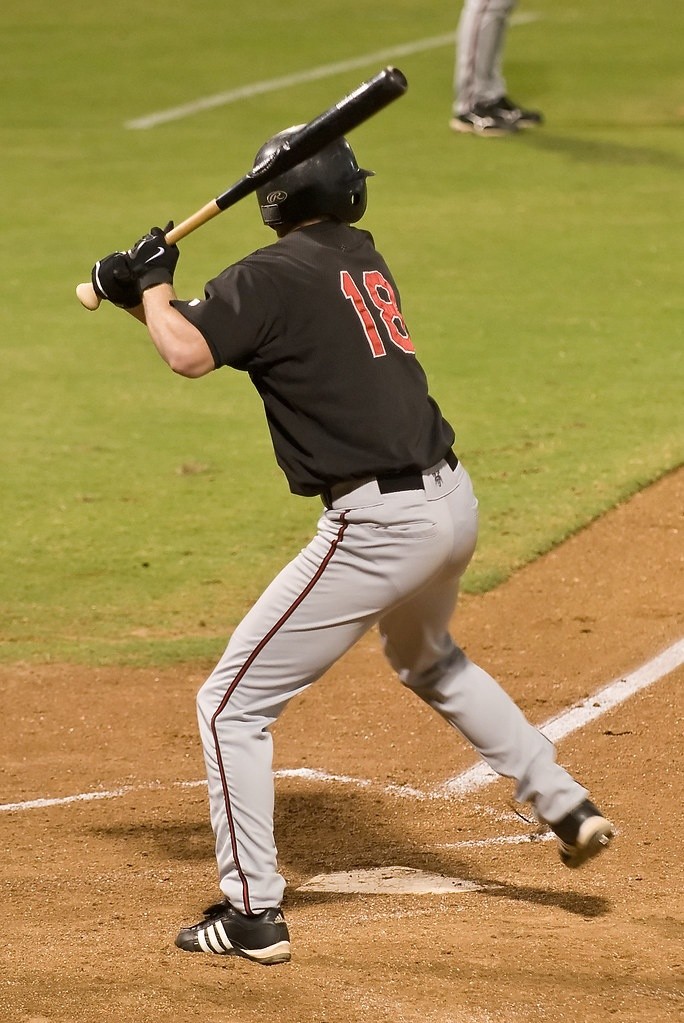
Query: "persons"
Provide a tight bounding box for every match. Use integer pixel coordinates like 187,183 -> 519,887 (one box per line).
92,122 -> 613,966
448,0 -> 545,138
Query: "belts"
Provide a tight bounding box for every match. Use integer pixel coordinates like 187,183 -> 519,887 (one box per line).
321,449 -> 458,510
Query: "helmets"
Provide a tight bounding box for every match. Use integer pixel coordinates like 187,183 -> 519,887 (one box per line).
253,123 -> 368,230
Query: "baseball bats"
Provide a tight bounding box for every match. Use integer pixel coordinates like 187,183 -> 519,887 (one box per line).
76,65 -> 410,311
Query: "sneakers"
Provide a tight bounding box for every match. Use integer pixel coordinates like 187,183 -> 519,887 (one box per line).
551,800 -> 612,869
175,907 -> 291,964
449,96 -> 541,137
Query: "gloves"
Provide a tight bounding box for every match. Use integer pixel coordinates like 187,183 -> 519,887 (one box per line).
90,253 -> 143,308
126,221 -> 179,292
